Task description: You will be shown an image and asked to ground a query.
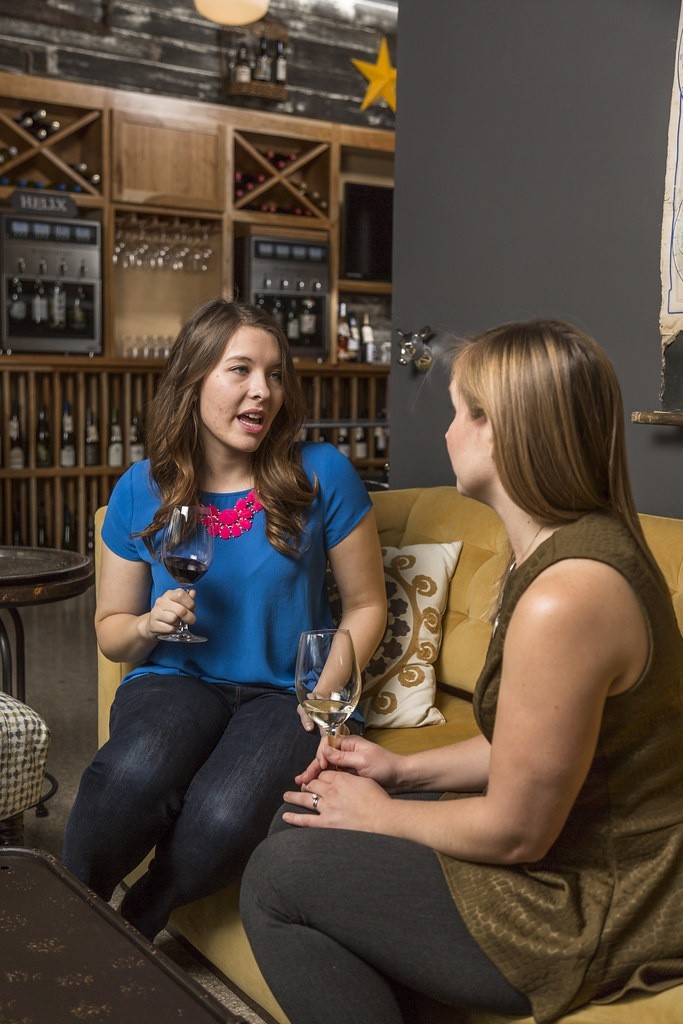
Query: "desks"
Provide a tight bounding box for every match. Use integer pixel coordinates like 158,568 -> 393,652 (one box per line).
0,546 -> 97,816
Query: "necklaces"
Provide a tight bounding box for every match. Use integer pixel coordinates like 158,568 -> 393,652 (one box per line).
491,525 -> 542,636
193,489 -> 264,540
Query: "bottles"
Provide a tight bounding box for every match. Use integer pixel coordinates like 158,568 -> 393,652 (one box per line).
61,406 -> 77,469
338,426 -> 350,460
84,417 -> 100,468
35,405 -> 53,467
255,274 -> 323,347
130,411 -> 145,466
7,257 -> 90,332
335,303 -> 376,361
376,426 -> 388,461
1,108 -> 101,194
114,333 -> 180,359
8,409 -> 25,469
320,427 -> 331,443
232,149 -> 329,217
355,426 -> 369,460
108,419 -> 123,467
230,35 -> 287,84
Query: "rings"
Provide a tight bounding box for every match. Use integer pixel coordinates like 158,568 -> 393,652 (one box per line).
313,794 -> 320,809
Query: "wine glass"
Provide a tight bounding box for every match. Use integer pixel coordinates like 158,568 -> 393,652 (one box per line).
114,217 -> 220,274
294,630 -> 362,771
157,504 -> 213,642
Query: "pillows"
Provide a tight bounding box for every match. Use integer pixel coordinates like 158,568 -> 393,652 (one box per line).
325,543 -> 464,730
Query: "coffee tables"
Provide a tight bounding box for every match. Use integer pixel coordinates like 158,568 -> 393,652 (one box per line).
0,846 -> 246,1024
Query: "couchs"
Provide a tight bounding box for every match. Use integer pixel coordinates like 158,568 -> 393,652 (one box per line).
96,489 -> 683,1024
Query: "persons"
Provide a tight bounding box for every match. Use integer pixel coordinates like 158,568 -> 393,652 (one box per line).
59,297 -> 389,941
237,321 -> 683,1024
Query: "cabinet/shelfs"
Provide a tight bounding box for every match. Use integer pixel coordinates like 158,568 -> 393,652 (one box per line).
0,70 -> 406,549
